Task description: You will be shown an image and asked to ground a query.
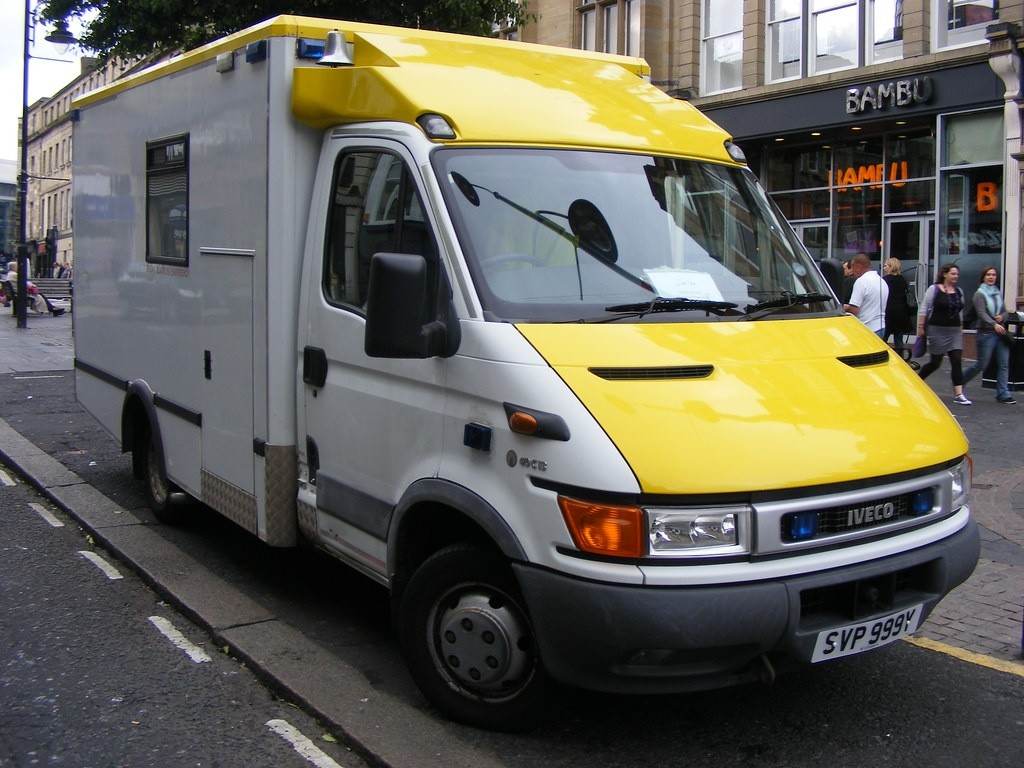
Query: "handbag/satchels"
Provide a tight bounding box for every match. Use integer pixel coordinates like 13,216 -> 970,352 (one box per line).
1001,330 -> 1016,346
903,277 -> 918,316
913,327 -> 926,358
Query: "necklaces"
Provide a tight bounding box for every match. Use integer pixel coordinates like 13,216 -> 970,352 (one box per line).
944,285 -> 960,319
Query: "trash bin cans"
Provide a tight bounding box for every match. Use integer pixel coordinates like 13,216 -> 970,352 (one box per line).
981,311 -> 1024,393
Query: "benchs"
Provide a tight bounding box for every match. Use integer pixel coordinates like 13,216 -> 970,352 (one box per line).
0,278 -> 73,316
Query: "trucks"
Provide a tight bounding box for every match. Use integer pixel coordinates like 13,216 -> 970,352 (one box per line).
70,15 -> 982,732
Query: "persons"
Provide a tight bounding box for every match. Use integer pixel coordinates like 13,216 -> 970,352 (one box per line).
843,254 -> 889,339
962,266 -> 1018,404
7,261 -> 65,317
841,260 -> 856,305
53,262 -> 73,278
916,263 -> 972,404
882,258 -> 912,358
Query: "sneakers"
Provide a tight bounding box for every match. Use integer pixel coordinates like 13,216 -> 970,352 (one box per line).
954,395 -> 972,405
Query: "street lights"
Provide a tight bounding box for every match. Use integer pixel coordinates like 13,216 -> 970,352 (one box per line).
15,0 -> 80,330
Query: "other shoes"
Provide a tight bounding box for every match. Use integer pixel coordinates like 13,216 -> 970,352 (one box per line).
1003,397 -> 1018,404
53,308 -> 66,317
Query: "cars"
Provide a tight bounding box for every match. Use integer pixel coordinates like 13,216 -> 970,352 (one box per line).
947,230 -> 1002,253
